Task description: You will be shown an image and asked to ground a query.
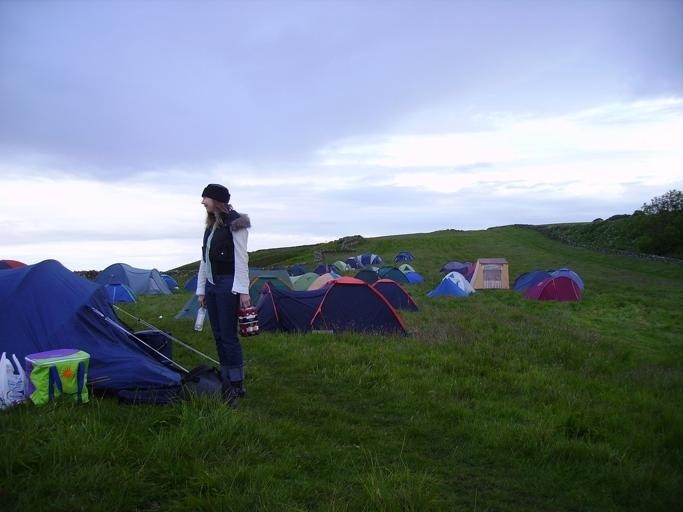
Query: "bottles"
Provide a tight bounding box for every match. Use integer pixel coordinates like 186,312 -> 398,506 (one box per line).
194,305 -> 205,332
237,306 -> 260,336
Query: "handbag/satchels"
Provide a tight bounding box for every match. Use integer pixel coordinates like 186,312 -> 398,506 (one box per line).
25,349 -> 90,406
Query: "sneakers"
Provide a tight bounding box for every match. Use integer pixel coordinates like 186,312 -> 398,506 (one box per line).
231,380 -> 246,396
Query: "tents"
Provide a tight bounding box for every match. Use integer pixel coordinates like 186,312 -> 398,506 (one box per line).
0,251 -> 584,399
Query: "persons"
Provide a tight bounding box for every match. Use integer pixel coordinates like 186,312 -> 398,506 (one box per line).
195,183 -> 250,398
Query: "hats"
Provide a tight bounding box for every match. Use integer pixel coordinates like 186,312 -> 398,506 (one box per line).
202,184 -> 230,203
